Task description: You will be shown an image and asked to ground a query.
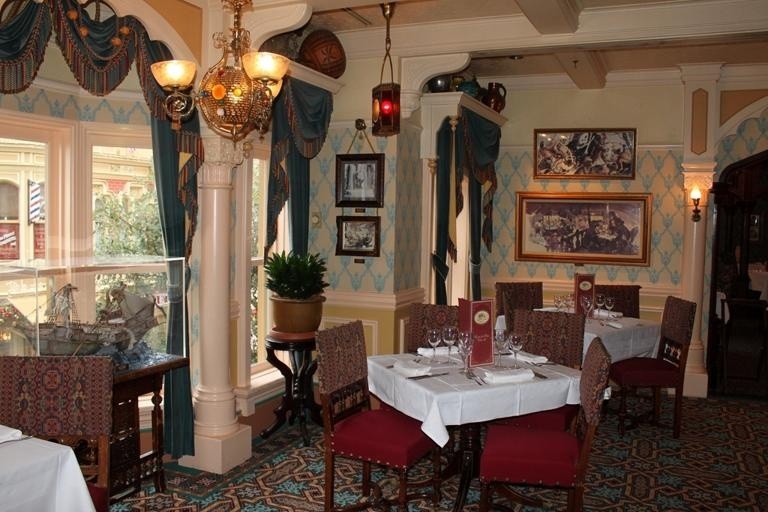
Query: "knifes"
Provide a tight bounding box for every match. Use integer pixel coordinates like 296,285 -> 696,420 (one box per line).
406,372 -> 450,380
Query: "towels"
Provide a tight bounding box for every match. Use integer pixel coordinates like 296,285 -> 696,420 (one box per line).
0,424 -> 22,444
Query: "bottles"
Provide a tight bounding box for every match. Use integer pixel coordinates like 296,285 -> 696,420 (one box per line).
453,76 -> 465,93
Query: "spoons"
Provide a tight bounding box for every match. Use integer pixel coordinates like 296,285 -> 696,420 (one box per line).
526,362 -> 558,366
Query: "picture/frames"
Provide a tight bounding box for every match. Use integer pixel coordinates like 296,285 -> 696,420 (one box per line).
336,153 -> 384,208
533,128 -> 637,180
335,216 -> 380,257
515,191 -> 650,267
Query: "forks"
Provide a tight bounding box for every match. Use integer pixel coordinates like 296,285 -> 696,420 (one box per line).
465,372 -> 487,386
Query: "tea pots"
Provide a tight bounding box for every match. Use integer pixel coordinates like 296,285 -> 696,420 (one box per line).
482,82 -> 508,113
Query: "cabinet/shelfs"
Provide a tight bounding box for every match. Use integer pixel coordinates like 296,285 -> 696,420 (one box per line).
706,151 -> 767,400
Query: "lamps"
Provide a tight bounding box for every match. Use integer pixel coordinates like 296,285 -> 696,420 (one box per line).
373,2 -> 401,137
691,184 -> 702,223
148,1 -> 291,151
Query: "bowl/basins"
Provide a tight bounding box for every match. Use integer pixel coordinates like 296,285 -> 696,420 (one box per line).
429,77 -> 451,91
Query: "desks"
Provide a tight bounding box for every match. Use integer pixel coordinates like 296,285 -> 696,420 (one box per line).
1,423 -> 97,512
259,326 -> 324,447
34,322 -> 189,507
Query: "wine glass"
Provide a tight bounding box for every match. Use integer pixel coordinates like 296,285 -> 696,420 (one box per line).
553,293 -> 616,321
428,325 -> 525,372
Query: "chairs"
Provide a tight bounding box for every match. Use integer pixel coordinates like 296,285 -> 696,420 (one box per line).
0,354 -> 113,512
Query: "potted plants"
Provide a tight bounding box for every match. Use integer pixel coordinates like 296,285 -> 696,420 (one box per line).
264,249 -> 330,343
